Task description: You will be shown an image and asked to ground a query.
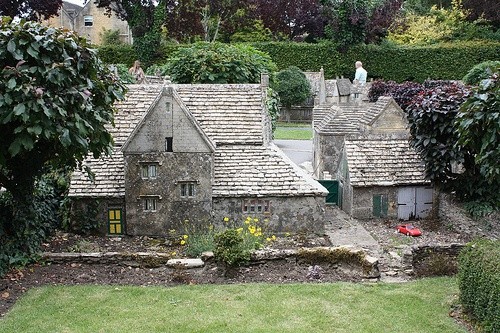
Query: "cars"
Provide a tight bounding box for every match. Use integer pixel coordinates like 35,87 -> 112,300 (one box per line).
396,223 -> 423,238
